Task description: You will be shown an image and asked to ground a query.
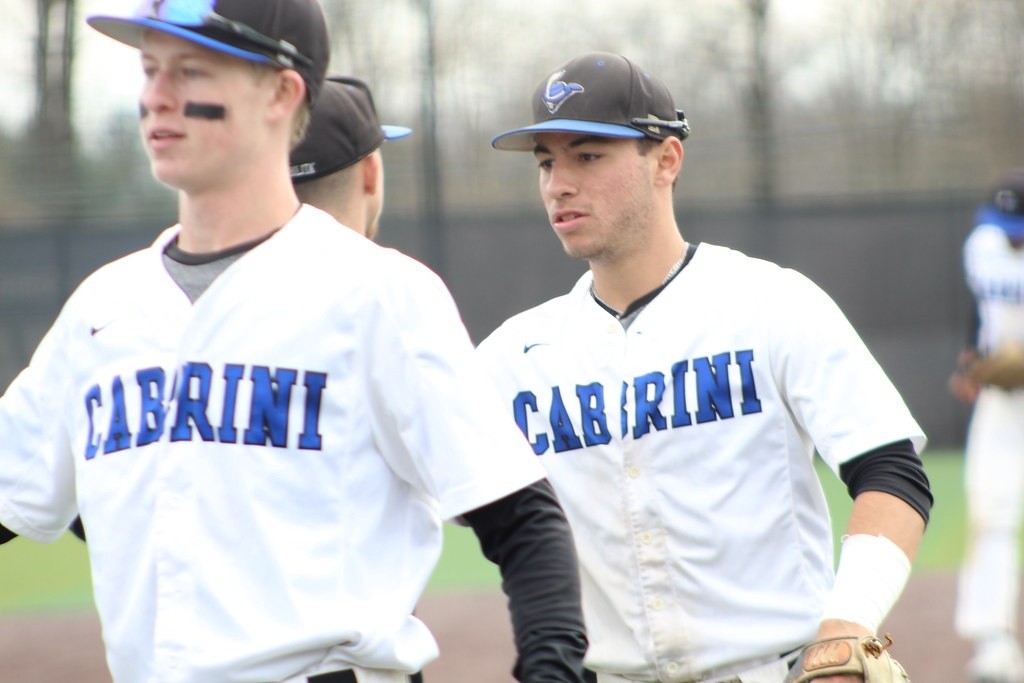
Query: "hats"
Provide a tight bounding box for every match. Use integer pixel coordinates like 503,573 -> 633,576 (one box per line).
86,0 -> 330,110
288,75 -> 410,184
490,52 -> 690,153
972,178 -> 1024,243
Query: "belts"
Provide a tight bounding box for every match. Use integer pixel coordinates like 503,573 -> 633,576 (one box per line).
306,665 -> 420,682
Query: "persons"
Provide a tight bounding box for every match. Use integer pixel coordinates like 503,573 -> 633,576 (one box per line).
288,74 -> 416,241
946,183 -> 1024,683
0,0 -> 600,683
477,53 -> 935,683
